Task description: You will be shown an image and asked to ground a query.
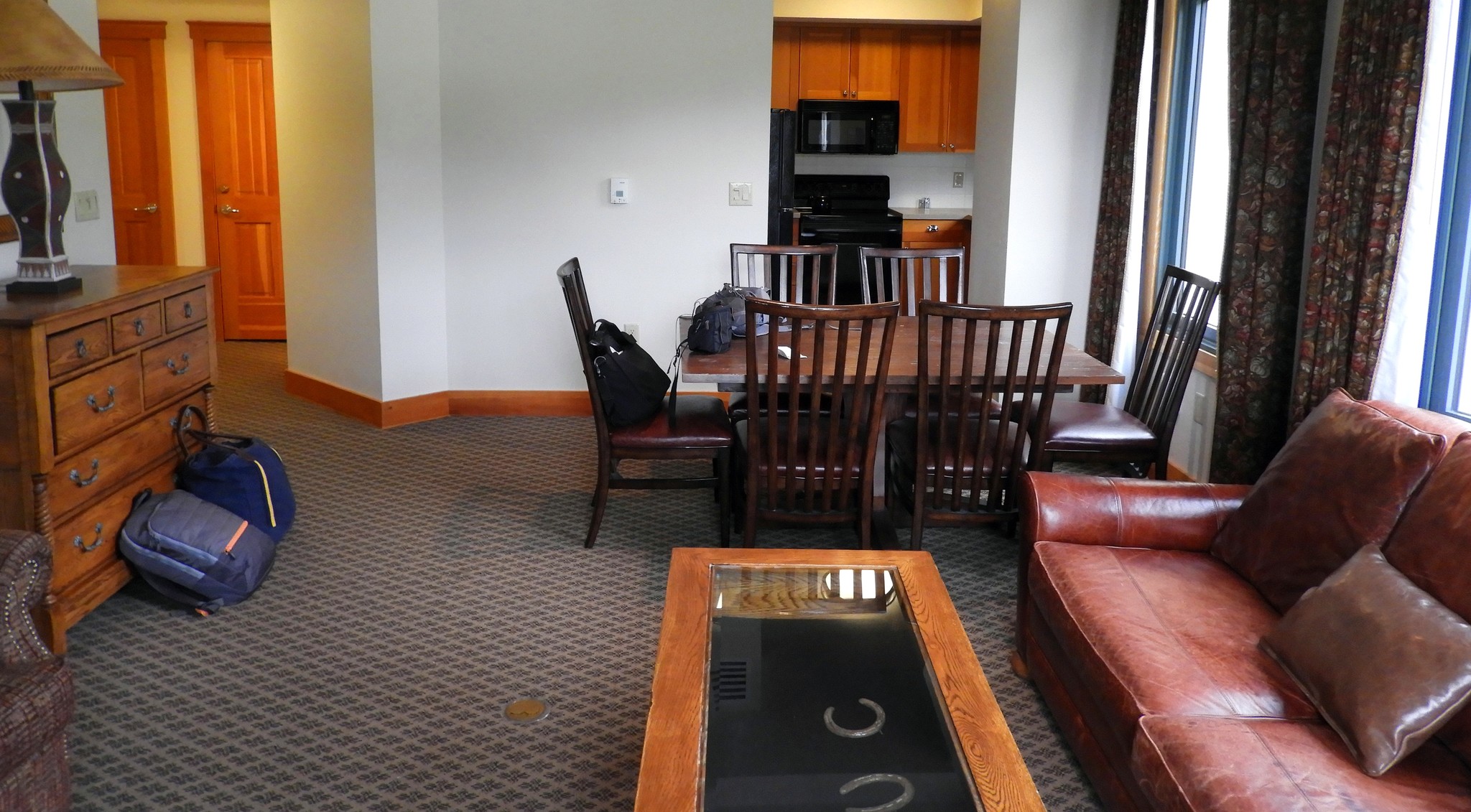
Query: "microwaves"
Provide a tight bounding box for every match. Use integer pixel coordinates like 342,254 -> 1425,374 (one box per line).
797,99 -> 899,155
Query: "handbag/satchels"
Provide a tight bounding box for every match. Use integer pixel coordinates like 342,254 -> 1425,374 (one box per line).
687,283 -> 774,355
119,486 -> 275,618
589,319 -> 671,427
175,402 -> 297,543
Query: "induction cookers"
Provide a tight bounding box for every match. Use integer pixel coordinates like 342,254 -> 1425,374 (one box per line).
793,173 -> 903,223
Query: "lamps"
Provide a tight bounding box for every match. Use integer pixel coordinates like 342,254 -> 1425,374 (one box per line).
0,0 -> 126,305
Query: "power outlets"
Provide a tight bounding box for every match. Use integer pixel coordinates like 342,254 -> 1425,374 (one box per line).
952,171 -> 965,189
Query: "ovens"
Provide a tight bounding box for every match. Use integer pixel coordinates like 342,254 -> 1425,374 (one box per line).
798,223 -> 902,306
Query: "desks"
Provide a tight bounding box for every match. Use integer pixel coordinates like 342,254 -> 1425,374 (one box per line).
675,308 -> 1129,515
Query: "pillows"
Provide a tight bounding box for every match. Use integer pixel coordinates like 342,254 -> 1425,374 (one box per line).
1255,426 -> 1471,781
1208,383 -> 1449,617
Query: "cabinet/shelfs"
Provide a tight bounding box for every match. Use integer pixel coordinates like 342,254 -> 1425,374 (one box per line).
0,262 -> 223,661
897,219 -> 974,316
770,19 -> 983,154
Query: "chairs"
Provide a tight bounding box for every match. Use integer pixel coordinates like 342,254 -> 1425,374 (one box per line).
554,239 -> 1222,555
0,522 -> 81,812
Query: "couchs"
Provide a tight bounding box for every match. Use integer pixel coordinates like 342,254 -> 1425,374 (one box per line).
1006,395 -> 1471,812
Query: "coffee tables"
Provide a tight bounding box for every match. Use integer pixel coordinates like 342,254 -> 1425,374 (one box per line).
630,538 -> 1053,812
